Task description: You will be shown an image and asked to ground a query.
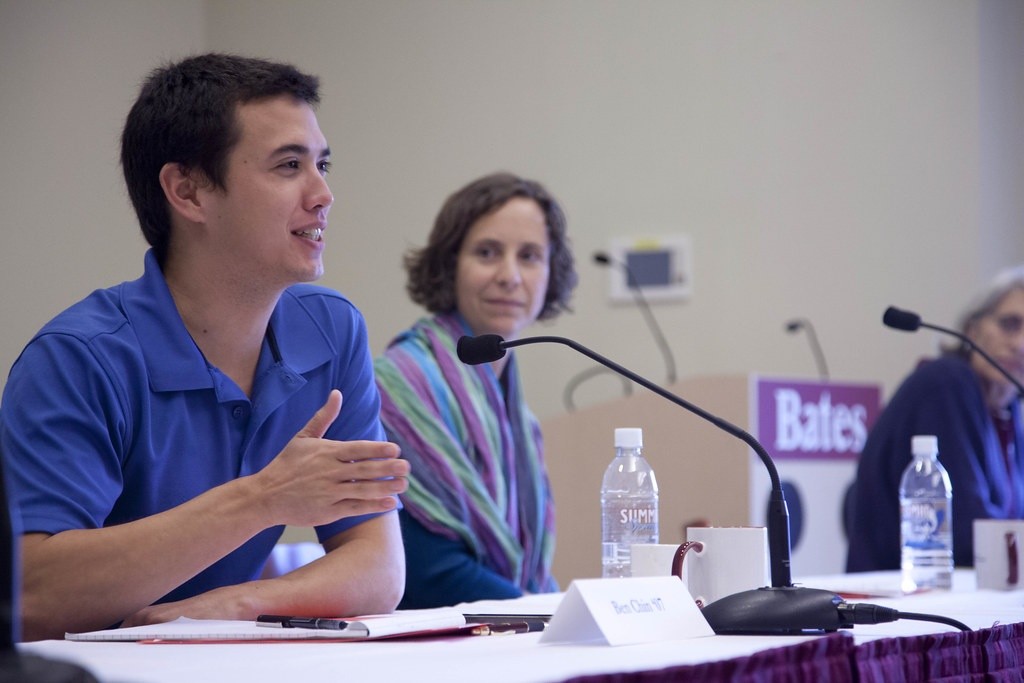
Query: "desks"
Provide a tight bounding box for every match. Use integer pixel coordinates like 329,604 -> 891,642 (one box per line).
0,570 -> 1024,683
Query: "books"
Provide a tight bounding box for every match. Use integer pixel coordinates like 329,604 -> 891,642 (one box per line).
449,590 -> 567,629
62,601 -> 493,644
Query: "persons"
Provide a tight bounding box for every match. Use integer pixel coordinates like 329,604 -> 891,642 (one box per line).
1,50 -> 413,647
372,172 -> 580,611
840,264 -> 1023,579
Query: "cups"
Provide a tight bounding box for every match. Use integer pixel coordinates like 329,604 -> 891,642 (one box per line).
671,527 -> 772,609
974,519 -> 1024,591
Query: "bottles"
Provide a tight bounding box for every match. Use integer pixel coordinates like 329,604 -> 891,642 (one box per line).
601,427 -> 659,577
899,434 -> 952,594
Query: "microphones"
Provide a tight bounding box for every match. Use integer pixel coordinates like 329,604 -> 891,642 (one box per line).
594,255 -> 676,382
883,308 -> 1024,394
785,320 -> 828,377
456,333 -> 854,632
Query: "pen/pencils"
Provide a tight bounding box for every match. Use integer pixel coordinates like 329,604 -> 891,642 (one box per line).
255,614 -> 351,631
